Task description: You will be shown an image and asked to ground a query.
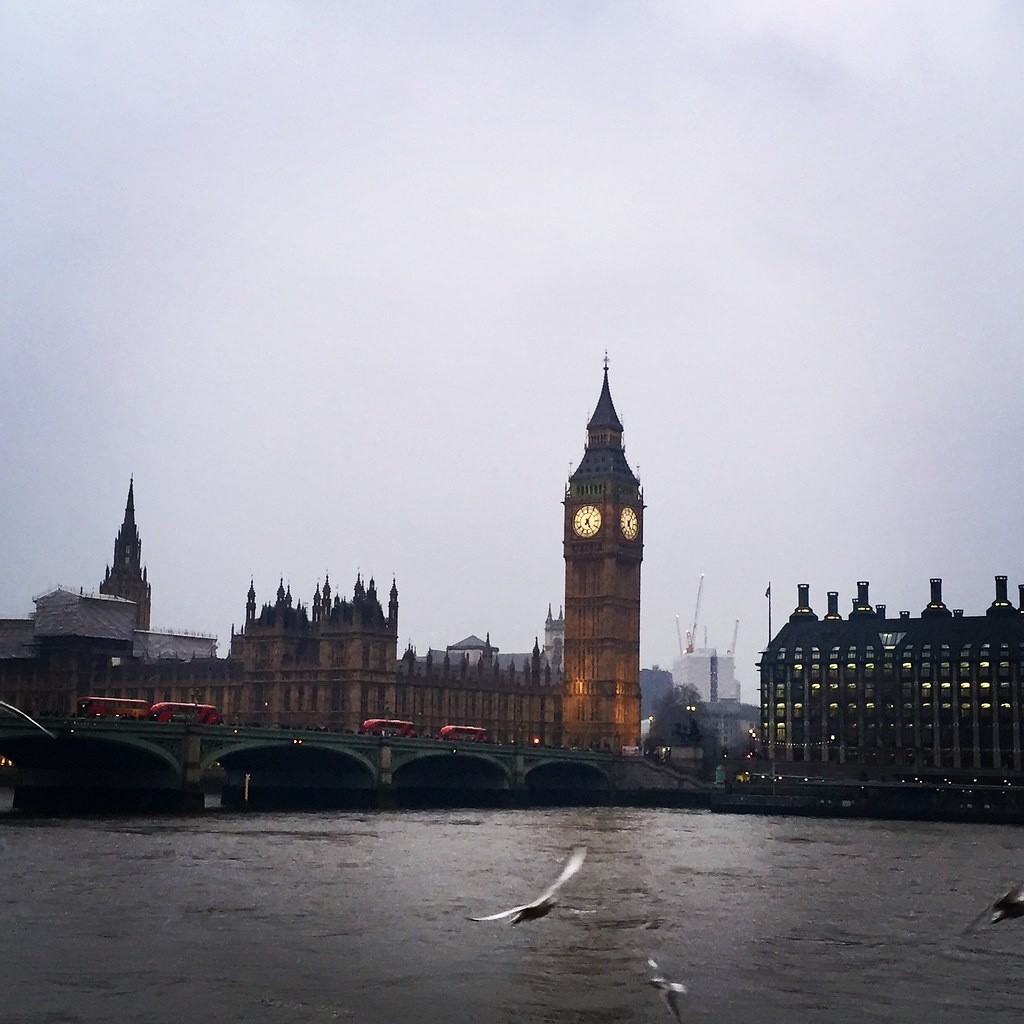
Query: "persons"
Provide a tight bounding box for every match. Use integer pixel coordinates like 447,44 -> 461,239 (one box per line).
211,717 -> 262,728
547,743 -> 611,753
167,716 -> 172,724
23,708 -> 65,718
358,727 -> 418,740
424,732 -> 505,744
71,712 -> 157,722
273,720 -> 354,735
646,746 -> 672,765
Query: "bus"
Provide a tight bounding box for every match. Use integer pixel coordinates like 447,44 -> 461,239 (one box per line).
438,726 -> 488,744
150,702 -> 222,724
76,696 -> 150,720
360,719 -> 418,739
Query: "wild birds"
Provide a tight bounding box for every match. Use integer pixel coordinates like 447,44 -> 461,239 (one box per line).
645,957 -> 689,1023
464,845 -> 587,925
989,879 -> 1024,925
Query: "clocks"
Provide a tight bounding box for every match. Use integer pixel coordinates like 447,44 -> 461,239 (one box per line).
571,504 -> 603,539
618,505 -> 640,542
